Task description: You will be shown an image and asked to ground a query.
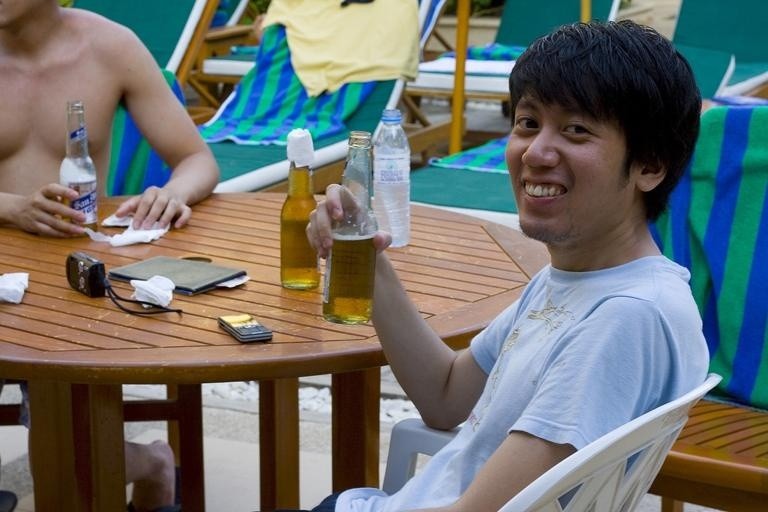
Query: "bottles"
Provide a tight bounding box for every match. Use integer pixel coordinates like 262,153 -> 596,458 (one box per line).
57,100 -> 98,237
322,131 -> 377,327
279,127 -> 321,292
371,108 -> 412,249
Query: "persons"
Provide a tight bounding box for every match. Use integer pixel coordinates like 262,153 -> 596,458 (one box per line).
0,0 -> 220,511
304,18 -> 711,512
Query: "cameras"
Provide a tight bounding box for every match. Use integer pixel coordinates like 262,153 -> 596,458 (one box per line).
66,251 -> 107,299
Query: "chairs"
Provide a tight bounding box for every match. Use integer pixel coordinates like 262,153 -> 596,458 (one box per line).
383,374 -> 720,512
0,70 -> 206,511
646,102 -> 768,512
74,1 -> 767,228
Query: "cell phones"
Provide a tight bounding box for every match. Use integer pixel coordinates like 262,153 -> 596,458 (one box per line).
216,313 -> 273,343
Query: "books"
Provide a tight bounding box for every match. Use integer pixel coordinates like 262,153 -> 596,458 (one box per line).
107,255 -> 251,296
